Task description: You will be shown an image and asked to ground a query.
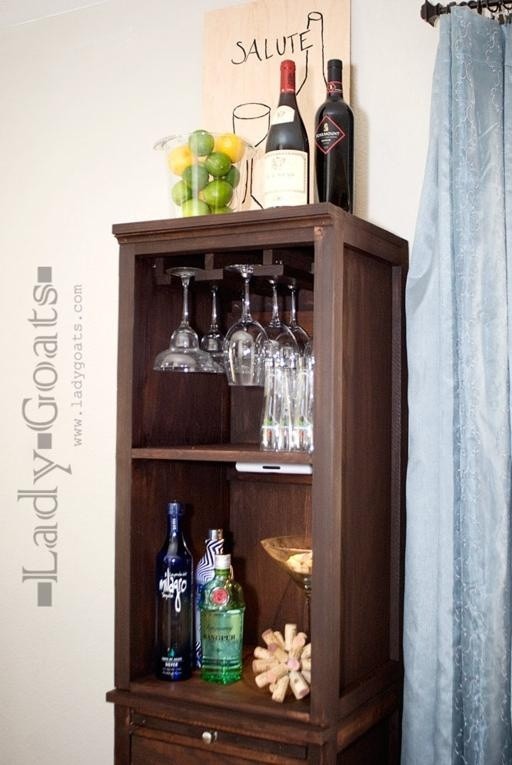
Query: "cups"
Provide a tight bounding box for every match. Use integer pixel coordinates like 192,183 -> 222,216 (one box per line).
153,131 -> 256,219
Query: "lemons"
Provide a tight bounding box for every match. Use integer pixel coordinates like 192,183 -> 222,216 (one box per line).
168,130 -> 244,218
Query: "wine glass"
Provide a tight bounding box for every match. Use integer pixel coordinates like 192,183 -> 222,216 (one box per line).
260,534 -> 312,642
153,263 -> 313,387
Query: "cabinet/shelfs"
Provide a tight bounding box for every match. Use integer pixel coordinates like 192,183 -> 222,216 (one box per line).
104,203 -> 409,763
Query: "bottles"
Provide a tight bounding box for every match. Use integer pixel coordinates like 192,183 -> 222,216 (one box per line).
314,59 -> 355,215
265,59 -> 310,208
152,499 -> 193,682
195,529 -> 234,669
199,552 -> 246,685
